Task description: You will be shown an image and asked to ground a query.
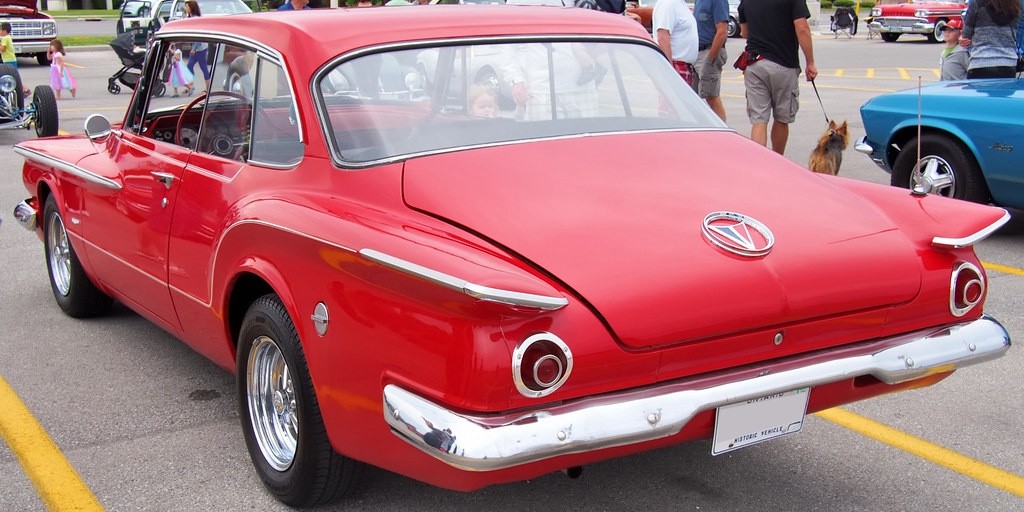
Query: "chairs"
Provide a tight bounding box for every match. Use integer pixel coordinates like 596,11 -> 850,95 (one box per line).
830,7 -> 859,39
863,10 -> 882,39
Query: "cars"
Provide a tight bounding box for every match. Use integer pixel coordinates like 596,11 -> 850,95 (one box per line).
115,0 -> 256,65
869,0 -> 969,44
853,77 -> 1024,215
401,0 -> 583,111
637,0 -> 741,38
0,0 -> 58,66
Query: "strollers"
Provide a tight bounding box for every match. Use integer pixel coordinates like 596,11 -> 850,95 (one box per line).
106,16 -> 178,99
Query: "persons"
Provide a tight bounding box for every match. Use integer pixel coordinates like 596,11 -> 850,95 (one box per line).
0,22 -> 31,98
47,39 -> 76,100
184,1 -> 210,95
166,41 -> 195,98
279,0 -> 1024,157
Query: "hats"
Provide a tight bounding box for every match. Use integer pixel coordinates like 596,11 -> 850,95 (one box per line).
939,17 -> 964,31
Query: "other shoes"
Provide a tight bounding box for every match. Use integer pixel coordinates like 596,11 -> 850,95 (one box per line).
188,87 -> 196,97
169,94 -> 180,98
24,89 -> 32,98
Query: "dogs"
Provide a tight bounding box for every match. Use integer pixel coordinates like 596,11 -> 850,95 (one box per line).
809,120 -> 850,176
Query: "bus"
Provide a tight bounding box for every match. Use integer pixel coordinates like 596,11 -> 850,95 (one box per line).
10,2 -> 1016,508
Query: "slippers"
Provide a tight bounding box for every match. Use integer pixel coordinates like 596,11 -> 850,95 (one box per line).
202,89 -> 208,94
182,90 -> 187,93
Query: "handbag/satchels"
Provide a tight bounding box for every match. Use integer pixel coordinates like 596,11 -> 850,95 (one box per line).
733,51 -> 766,71
1016,56 -> 1024,72
